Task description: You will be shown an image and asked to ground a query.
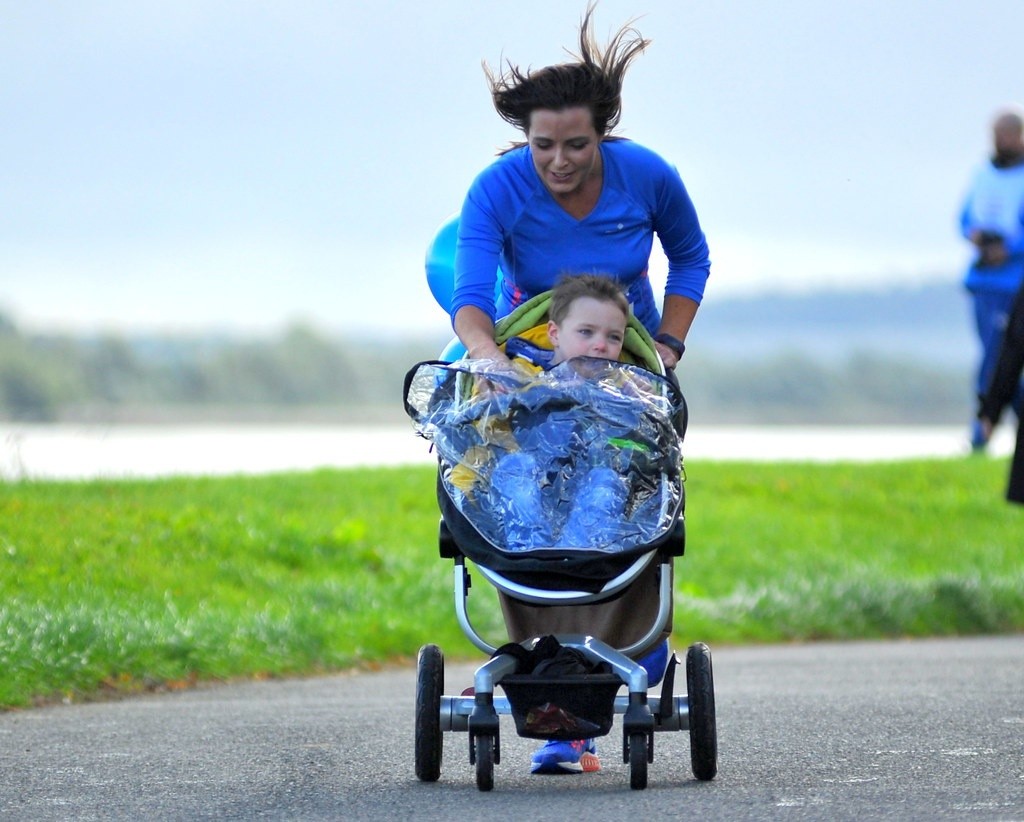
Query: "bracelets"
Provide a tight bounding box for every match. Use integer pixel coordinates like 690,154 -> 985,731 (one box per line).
656,333 -> 687,361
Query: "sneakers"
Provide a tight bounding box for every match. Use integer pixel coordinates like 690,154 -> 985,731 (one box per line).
637,636 -> 668,689
528,736 -> 602,775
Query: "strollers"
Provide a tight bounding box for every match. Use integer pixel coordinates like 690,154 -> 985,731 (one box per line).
404,289 -> 720,796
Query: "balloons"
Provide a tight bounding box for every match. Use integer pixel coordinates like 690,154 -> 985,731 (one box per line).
437,335 -> 471,388
426,209 -> 505,319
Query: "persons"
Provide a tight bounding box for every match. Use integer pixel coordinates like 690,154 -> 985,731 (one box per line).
959,113 -> 1024,452
450,0 -> 715,775
979,281 -> 1024,510
487,269 -> 653,567
